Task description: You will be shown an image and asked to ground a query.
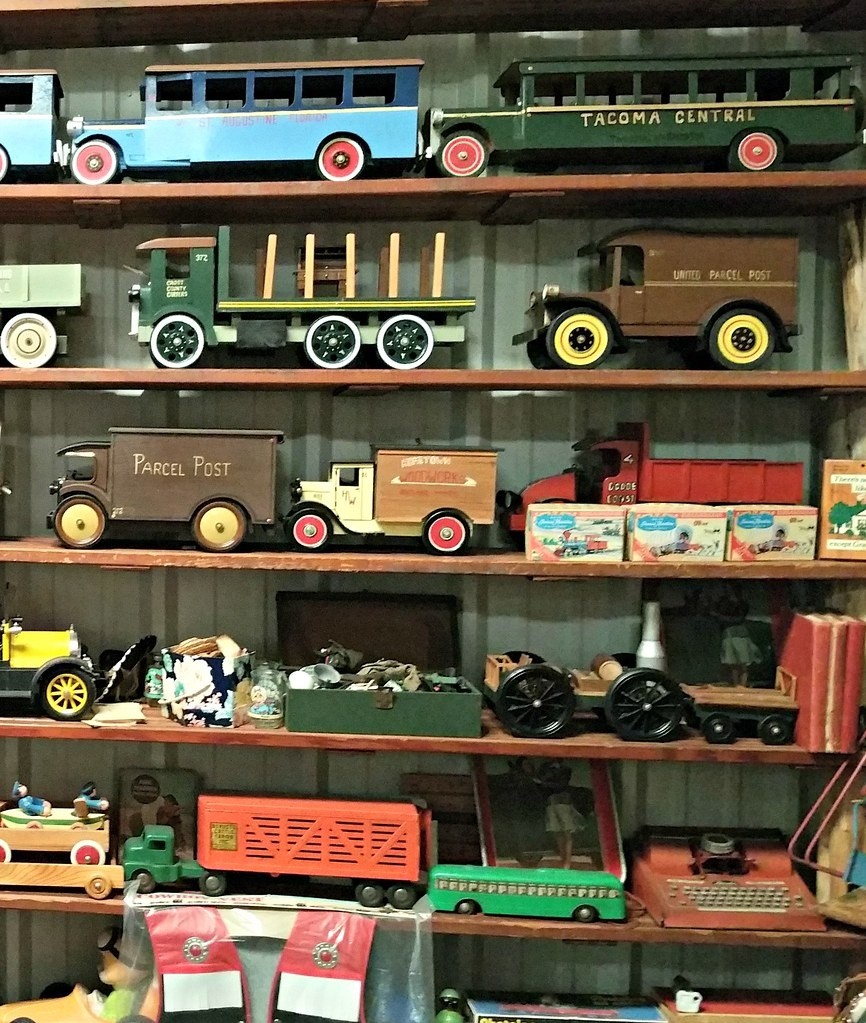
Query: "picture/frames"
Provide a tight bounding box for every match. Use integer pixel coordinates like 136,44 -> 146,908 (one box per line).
468,756 -> 627,885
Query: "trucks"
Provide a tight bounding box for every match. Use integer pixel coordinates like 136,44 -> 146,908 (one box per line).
127,229 -> 478,369
0,264 -> 85,370
276,444 -> 505,557
512,226 -> 806,370
495,422 -> 804,552
44,427 -> 286,551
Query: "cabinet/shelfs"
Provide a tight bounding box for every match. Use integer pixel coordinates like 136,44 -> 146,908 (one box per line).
0,1 -> 866,1023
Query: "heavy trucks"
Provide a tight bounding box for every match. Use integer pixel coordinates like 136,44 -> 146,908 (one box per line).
121,794 -> 421,910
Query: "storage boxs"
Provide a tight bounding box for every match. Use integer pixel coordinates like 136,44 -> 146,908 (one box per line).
821,459 -> 866,560
727,504 -> 820,560
524,499 -> 626,563
626,501 -> 727,560
274,589 -> 482,740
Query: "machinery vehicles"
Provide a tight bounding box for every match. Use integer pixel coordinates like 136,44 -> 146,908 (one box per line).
0,616 -> 97,723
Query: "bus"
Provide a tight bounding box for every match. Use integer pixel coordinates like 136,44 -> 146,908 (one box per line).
0,70 -> 65,182
63,57 -> 427,184
427,864 -> 626,924
425,50 -> 866,178
